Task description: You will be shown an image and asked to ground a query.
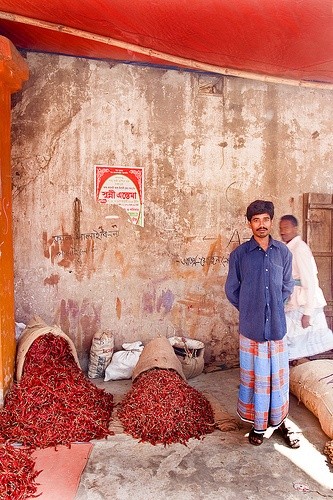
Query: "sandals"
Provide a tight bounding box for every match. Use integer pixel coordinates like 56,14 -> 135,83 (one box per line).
278,422 -> 300,449
249,427 -> 263,446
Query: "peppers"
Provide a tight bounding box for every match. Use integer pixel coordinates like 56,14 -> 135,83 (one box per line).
117,368 -> 220,447
0,332 -> 117,500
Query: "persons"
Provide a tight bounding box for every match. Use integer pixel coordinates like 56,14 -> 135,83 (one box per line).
279,215 -> 333,366
225,200 -> 301,448
